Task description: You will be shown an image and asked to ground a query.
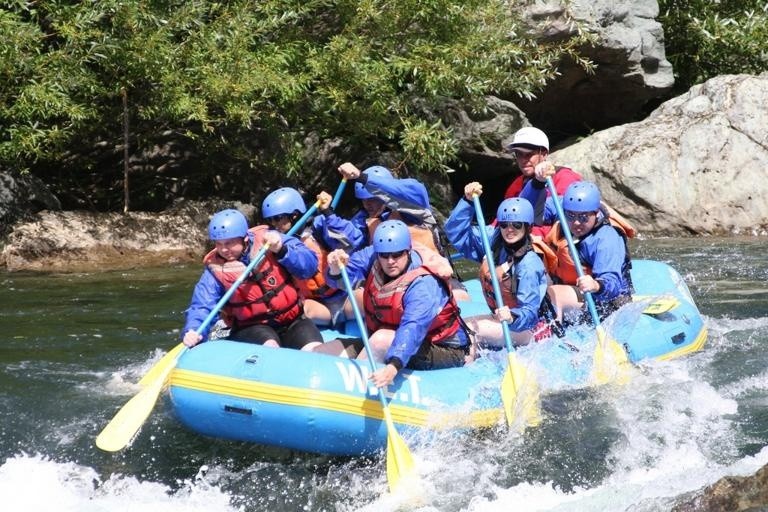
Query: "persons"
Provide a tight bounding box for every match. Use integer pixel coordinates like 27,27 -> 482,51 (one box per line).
180,209 -> 325,352
338,162 -> 470,301
443,181 -> 548,365
490,126 -> 585,243
325,219 -> 476,389
518,161 -> 636,330
261,186 -> 367,331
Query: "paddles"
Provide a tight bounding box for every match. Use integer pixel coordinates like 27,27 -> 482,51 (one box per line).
544,166 -> 629,385
629,293 -> 679,313
95,240 -> 271,452
337,260 -> 415,494
472,194 -> 542,429
134,200 -> 322,396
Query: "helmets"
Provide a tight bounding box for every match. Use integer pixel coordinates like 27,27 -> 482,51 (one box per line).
563,181 -> 601,212
506,127 -> 549,155
208,209 -> 248,241
355,166 -> 393,200
373,219 -> 412,252
496,197 -> 535,226
262,187 -> 307,220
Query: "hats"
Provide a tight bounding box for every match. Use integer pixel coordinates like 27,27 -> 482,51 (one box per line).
511,147 -> 533,154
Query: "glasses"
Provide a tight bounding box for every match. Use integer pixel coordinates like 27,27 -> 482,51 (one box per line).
267,216 -> 280,224
379,250 -> 404,259
565,215 -> 588,223
499,222 -> 522,229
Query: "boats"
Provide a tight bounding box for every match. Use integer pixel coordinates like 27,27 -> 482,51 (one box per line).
164,257 -> 709,462
